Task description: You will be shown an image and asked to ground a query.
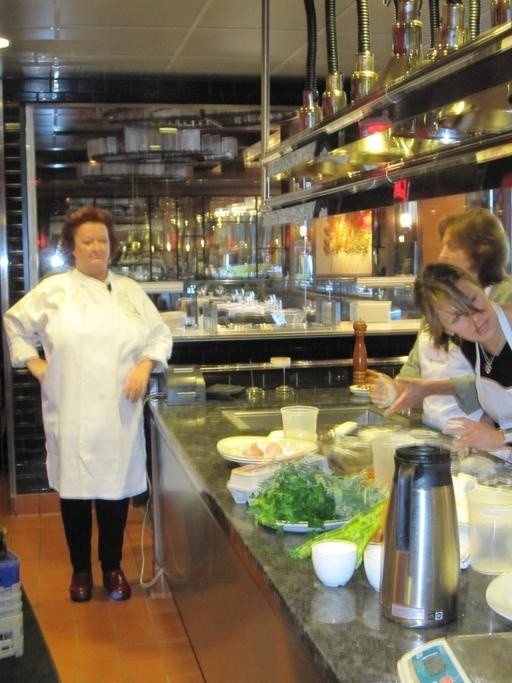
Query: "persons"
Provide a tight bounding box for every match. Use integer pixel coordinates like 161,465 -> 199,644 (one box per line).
362,208 -> 512,436
412,262 -> 512,452
2,205 -> 173,602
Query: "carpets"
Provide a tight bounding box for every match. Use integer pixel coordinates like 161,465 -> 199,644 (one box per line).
0,582 -> 61,682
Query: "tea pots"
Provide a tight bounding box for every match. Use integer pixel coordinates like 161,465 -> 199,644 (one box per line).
380,443 -> 461,631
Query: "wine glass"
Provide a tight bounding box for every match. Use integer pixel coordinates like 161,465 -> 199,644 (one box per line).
184,284 -> 282,334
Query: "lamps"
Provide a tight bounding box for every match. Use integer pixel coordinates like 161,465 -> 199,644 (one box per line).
74,128 -> 239,185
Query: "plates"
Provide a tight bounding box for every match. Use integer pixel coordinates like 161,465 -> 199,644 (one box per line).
349,385 -> 374,398
485,571 -> 512,621
216,429 -> 349,532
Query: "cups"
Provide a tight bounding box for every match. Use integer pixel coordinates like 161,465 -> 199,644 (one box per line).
372,432 -> 416,482
363,544 -> 383,592
465,487 -> 511,574
280,405 -> 320,441
311,540 -> 357,587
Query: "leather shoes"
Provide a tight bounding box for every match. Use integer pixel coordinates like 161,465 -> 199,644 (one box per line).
103,570 -> 130,600
69,569 -> 91,601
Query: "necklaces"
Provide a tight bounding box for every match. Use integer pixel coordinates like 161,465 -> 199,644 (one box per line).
480,334 -> 503,374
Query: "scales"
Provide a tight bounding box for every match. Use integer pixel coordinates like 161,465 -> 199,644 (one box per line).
397,632 -> 512,683
